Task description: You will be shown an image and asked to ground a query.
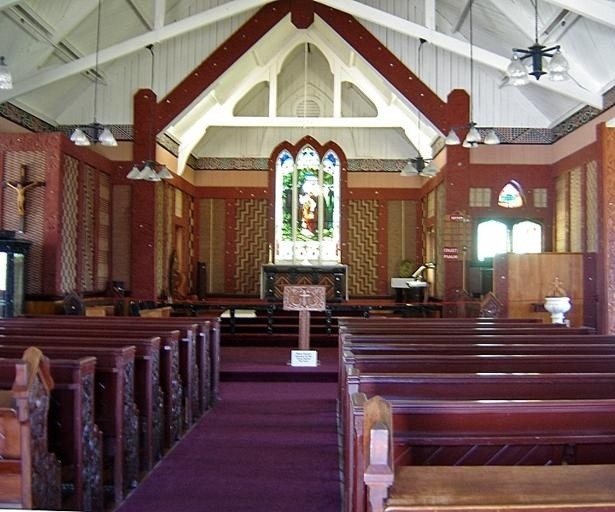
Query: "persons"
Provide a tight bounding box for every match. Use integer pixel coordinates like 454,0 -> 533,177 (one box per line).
6,181 -> 40,216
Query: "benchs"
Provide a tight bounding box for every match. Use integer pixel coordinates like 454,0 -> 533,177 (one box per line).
0,314 -> 222,510
335,317 -> 614,510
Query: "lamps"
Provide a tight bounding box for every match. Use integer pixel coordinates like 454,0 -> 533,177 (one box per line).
0,56 -> 14,91
495,0 -> 571,90
66,0 -> 176,183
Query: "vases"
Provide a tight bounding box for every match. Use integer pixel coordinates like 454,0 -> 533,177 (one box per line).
543,295 -> 572,323
